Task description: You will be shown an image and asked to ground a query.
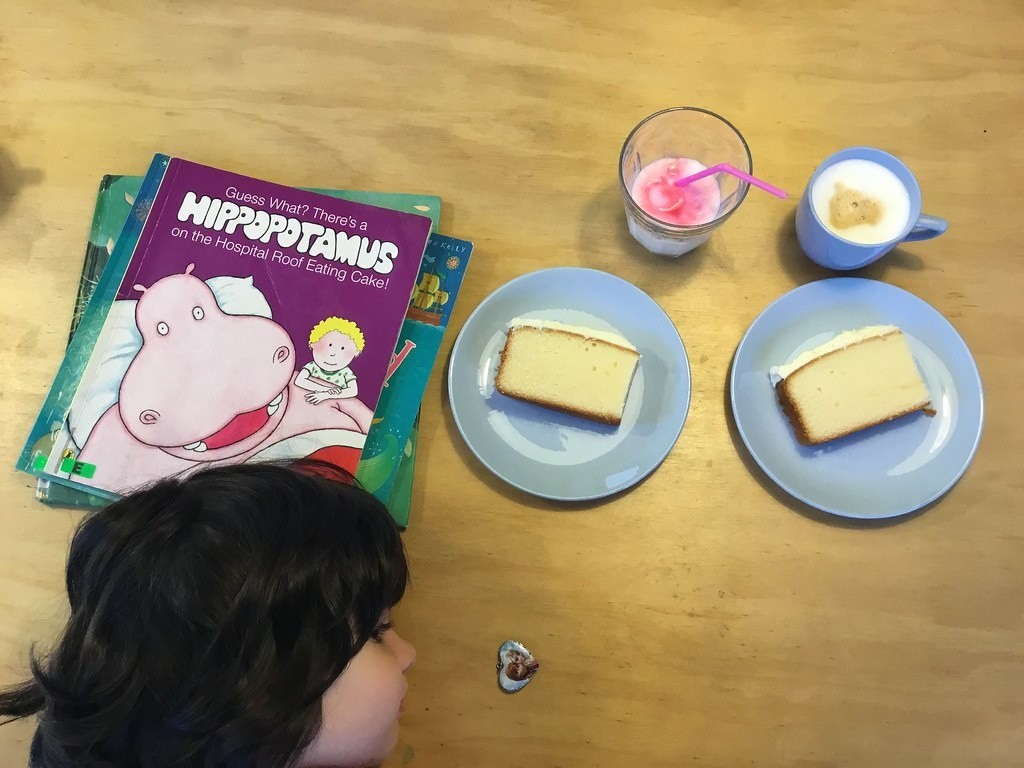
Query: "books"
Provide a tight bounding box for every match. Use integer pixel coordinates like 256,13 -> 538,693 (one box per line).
14,153 -> 474,528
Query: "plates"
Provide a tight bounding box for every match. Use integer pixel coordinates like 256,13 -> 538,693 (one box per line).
729,276 -> 985,520
447,266 -> 692,502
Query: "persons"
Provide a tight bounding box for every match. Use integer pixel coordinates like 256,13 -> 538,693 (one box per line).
0,462 -> 417,768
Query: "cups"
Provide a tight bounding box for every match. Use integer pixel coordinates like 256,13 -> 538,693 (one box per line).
795,147 -> 949,271
618,107 -> 753,260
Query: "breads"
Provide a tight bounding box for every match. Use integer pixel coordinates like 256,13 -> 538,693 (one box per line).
776,328 -> 932,446
494,324 -> 641,426
